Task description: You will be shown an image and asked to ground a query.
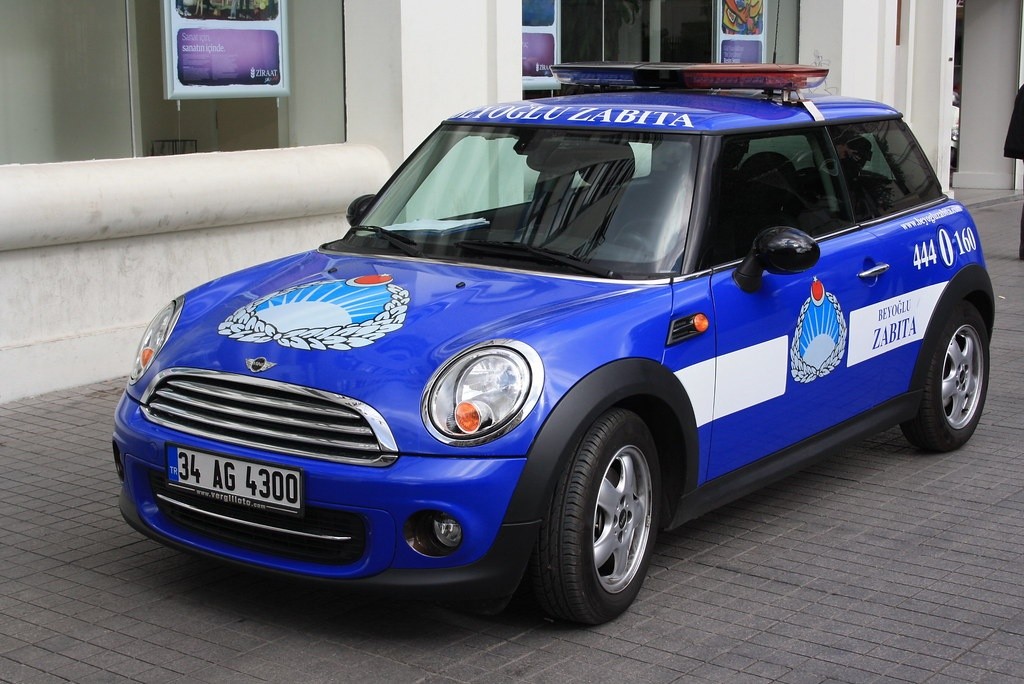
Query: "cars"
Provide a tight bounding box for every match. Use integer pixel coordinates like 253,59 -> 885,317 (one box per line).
112,89 -> 996,626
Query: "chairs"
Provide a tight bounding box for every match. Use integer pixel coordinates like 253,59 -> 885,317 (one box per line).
705,152 -> 799,271
518,143 -> 636,256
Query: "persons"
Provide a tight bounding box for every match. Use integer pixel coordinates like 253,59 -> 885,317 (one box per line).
1004,83 -> 1024,260
